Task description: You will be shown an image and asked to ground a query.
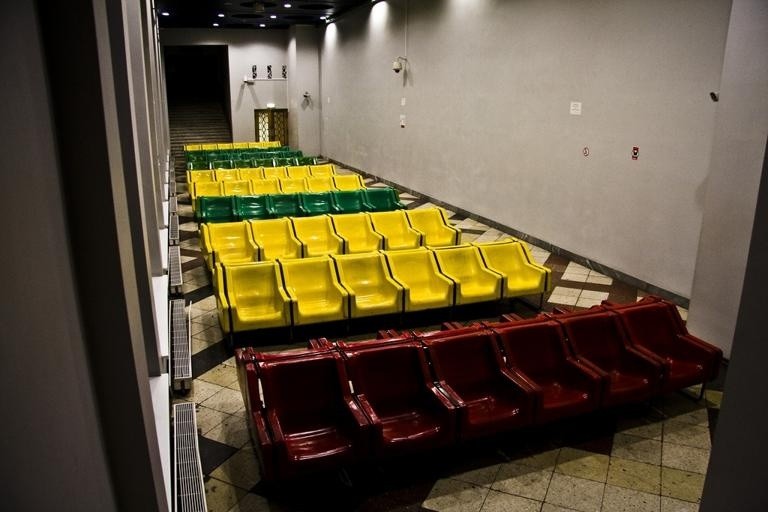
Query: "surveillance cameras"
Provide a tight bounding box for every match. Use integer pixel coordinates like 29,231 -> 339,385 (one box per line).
392,61 -> 402,73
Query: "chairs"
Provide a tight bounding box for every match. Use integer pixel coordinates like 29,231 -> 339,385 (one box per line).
236,292 -> 722,482
184,141 -> 555,337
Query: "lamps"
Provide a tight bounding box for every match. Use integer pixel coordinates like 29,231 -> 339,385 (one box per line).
318,5 -> 337,27
393,56 -> 407,73
243,74 -> 254,85
